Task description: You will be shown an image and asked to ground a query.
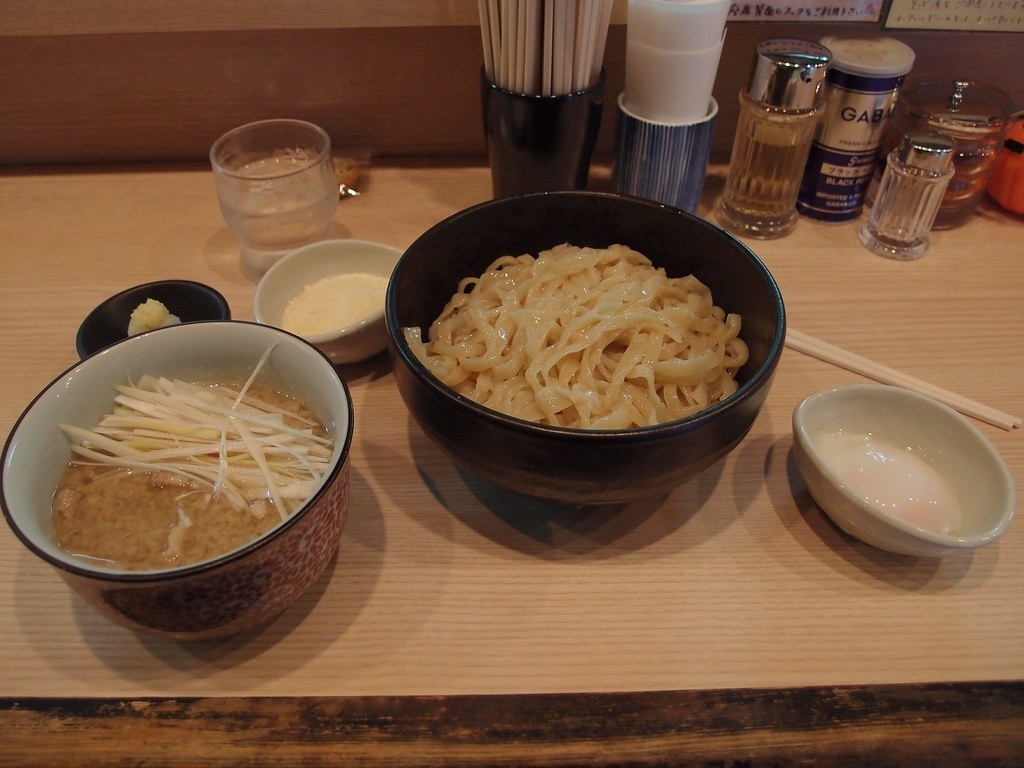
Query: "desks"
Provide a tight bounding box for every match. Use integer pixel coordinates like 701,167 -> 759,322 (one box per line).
0,147 -> 1024,768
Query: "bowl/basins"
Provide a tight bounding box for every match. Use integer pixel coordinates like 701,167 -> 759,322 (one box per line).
1,321 -> 353,639
385,191 -> 787,505
252,239 -> 407,367
76,278 -> 233,363
793,384 -> 1013,554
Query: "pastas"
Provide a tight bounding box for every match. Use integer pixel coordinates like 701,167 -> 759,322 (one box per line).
397,244 -> 748,433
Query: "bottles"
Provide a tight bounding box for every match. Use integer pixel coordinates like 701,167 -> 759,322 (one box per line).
864,73 -> 1011,231
715,38 -> 833,240
857,129 -> 956,261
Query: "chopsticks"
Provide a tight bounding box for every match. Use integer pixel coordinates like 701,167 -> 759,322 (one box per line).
478,0 -> 613,95
784,328 -> 1023,431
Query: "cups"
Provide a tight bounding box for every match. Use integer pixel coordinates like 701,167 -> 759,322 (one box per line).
612,91 -> 718,216
479,65 -> 606,200
210,119 -> 342,279
627,38 -> 724,121
627,1 -> 731,51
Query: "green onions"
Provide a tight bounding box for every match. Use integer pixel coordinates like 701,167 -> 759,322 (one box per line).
55,343 -> 334,526
